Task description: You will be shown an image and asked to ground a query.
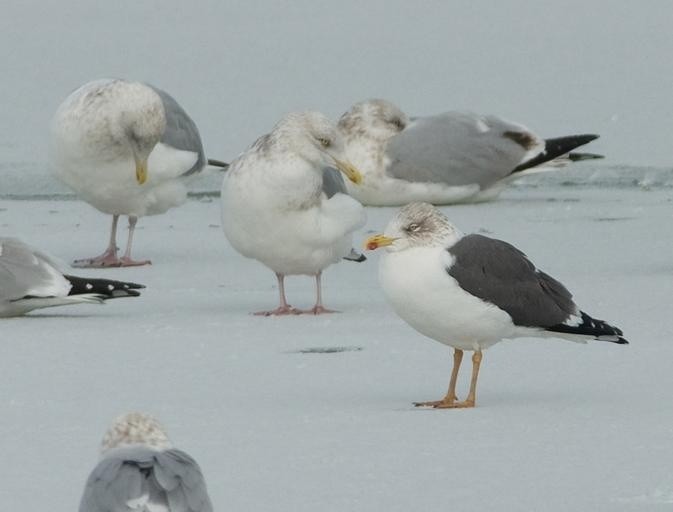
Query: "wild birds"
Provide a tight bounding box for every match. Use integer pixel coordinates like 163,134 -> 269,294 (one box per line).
220,110 -> 367,317
77,410 -> 214,512
0,236 -> 147,319
44,77 -> 231,269
362,201 -> 629,409
334,99 -> 605,207
